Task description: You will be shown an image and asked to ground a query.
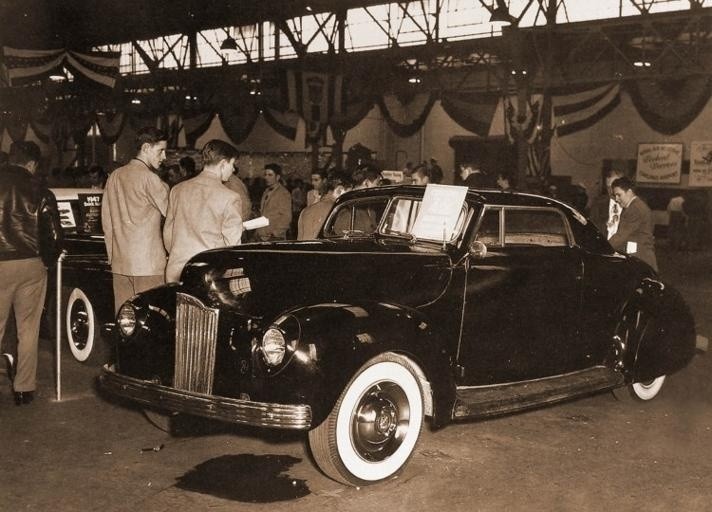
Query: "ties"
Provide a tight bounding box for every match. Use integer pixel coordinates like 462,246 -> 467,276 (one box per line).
612,204 -> 619,223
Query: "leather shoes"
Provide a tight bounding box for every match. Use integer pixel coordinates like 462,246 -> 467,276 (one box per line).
14,392 -> 33,405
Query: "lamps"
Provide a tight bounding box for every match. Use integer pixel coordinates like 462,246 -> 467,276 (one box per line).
489,0 -> 512,27
220,24 -> 238,50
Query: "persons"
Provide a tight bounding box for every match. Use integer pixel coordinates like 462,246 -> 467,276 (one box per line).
460,156 -> 518,189
297,159 -> 443,241
590,169 -> 660,274
1,140 -> 61,405
101,126 -> 171,324
83,165 -> 108,189
163,139 -> 246,285
157,157 -> 292,243
105,161 -> 119,175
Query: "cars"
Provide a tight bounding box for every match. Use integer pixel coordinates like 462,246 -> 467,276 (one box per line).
95,184 -> 699,489
6,184 -> 119,364
